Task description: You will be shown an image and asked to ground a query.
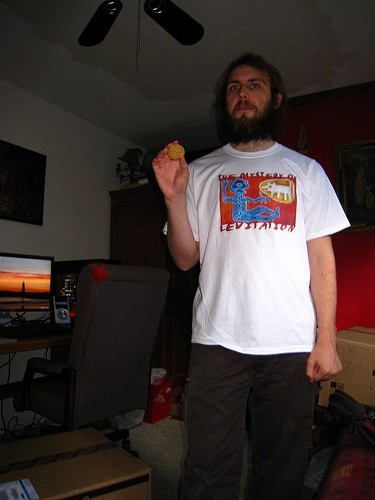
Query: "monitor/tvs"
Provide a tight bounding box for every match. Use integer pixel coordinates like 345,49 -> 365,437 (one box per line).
0,251 -> 55,311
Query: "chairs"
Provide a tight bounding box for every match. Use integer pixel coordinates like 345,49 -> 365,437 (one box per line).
12,261 -> 171,425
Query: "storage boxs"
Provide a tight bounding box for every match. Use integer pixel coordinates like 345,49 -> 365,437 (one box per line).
317,326 -> 375,408
0,427 -> 152,500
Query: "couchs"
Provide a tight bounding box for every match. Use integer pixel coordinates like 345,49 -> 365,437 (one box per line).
318,440 -> 375,500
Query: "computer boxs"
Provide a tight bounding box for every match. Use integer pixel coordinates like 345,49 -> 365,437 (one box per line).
52,259 -> 122,328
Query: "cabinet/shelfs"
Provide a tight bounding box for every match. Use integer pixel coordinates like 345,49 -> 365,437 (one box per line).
109,183 -> 168,261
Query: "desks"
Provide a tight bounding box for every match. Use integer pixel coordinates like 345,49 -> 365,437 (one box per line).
0,328 -> 71,354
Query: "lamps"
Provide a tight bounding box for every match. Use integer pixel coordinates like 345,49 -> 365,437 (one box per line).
77,0 -> 205,47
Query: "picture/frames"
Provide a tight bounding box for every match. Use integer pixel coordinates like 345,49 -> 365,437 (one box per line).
0,139 -> 48,226
333,139 -> 375,232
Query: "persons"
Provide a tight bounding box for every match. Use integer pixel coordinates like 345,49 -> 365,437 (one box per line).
152,51 -> 351,500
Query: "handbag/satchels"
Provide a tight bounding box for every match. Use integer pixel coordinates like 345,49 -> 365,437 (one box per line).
143,377 -> 173,424
